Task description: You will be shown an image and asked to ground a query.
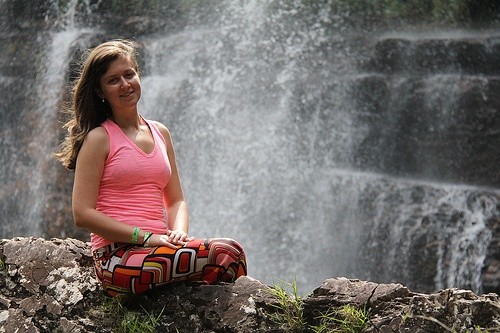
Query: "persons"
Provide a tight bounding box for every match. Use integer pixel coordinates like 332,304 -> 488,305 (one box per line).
53,40 -> 248,303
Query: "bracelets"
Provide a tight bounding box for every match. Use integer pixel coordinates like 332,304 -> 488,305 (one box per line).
139,233 -> 154,246
143,232 -> 152,247
131,226 -> 141,245
